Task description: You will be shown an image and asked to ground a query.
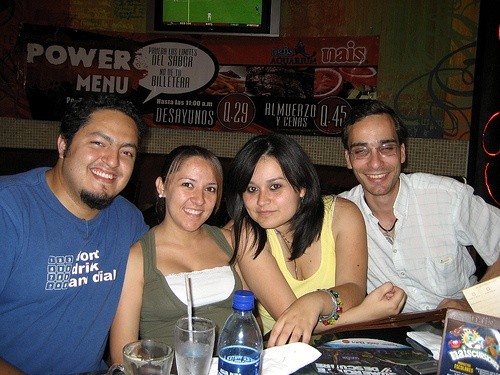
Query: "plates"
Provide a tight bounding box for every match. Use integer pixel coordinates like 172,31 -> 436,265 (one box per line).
218,77 -> 257,100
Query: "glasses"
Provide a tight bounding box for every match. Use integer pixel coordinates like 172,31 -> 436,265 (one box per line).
348,142 -> 401,159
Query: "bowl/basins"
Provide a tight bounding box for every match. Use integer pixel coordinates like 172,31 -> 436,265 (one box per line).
336,65 -> 377,86
312,67 -> 344,100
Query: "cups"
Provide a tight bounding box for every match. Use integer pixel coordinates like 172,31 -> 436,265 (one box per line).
174,316 -> 216,375
106,339 -> 174,375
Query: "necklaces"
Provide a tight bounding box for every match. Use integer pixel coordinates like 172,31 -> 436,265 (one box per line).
364,194 -> 397,244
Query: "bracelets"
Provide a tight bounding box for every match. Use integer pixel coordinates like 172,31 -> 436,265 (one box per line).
315,289 -> 343,326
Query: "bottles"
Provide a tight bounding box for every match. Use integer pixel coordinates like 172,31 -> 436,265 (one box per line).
218,290 -> 263,375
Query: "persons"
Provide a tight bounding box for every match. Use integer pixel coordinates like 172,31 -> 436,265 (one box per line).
108,146 -> 264,375
226,134 -> 408,347
0,93 -> 152,375
219,100 -> 500,313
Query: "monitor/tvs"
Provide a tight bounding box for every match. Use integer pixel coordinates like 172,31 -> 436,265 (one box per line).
146,0 -> 280,38
